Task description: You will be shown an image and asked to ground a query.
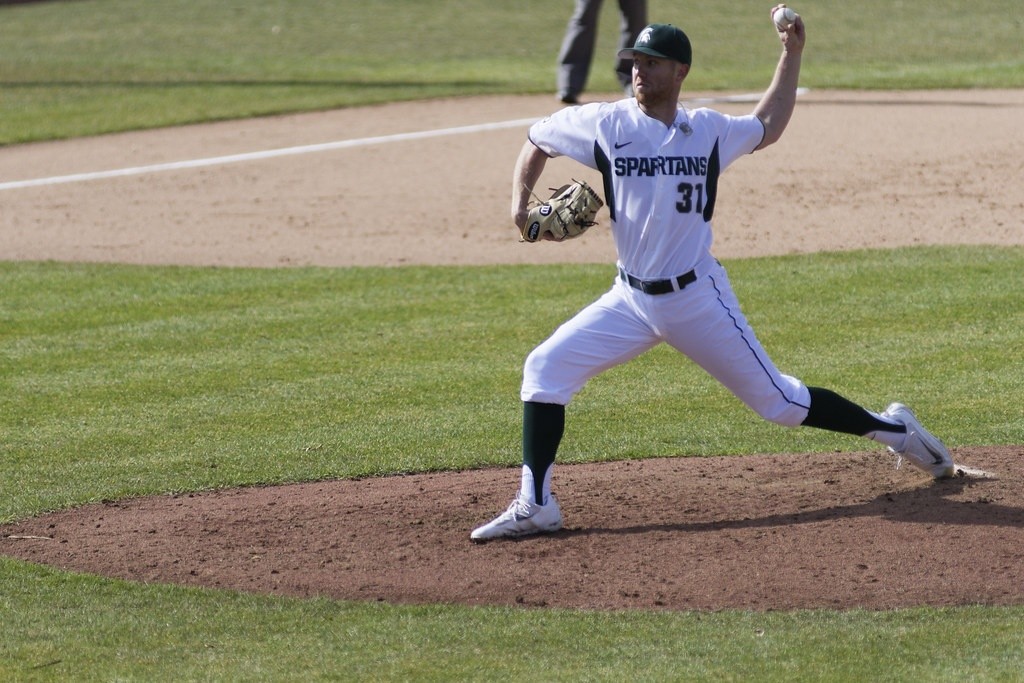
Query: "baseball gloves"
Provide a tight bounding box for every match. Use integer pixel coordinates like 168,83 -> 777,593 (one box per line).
518,178 -> 605,243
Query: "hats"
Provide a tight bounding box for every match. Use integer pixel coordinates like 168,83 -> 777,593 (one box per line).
617,23 -> 692,66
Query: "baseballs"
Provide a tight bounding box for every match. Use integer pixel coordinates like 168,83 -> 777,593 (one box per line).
774,7 -> 795,30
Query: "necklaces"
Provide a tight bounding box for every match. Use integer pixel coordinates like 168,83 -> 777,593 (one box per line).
641,98 -> 694,138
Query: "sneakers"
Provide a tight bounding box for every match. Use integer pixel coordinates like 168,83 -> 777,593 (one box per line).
470,495 -> 564,543
881,403 -> 955,478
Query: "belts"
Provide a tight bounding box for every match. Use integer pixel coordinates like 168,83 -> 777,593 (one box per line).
620,268 -> 697,295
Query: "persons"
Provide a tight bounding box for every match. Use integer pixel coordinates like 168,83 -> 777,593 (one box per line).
469,3 -> 953,543
554,0 -> 653,103
